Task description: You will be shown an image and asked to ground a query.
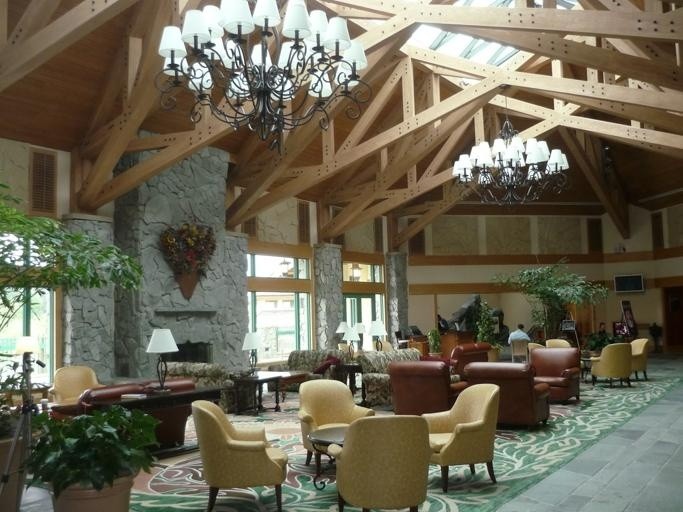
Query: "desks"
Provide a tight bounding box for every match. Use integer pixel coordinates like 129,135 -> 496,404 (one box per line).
82,388 -> 227,459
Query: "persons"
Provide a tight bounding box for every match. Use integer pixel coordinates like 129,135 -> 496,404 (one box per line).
438,314 -> 450,334
508,323 -> 530,344
596,323 -> 609,336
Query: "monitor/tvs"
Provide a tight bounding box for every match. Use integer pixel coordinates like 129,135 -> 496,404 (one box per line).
614,274 -> 644,293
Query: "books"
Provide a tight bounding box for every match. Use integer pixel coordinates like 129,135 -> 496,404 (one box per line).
121,394 -> 147,400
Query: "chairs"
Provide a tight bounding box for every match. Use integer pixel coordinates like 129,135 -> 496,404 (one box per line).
49,366 -> 107,402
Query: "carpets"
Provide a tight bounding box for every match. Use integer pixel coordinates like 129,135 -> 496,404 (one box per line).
20,356 -> 683,510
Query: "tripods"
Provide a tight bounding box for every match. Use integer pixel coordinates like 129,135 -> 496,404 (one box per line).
0,359 -> 53,512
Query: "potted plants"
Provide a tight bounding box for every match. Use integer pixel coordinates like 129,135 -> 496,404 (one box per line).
426,331 -> 443,358
19,404 -> 162,512
0,182 -> 143,511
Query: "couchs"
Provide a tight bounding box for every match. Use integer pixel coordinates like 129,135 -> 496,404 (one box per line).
50,377 -> 196,452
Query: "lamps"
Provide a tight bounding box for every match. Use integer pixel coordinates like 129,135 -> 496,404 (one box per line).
350,264 -> 363,277
280,259 -> 291,272
155,1 -> 373,153
145,329 -> 180,396
336,321 -> 388,363
452,84 -> 569,206
14,335 -> 41,404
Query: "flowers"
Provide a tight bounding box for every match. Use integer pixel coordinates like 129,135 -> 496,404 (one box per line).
160,223 -> 217,279
